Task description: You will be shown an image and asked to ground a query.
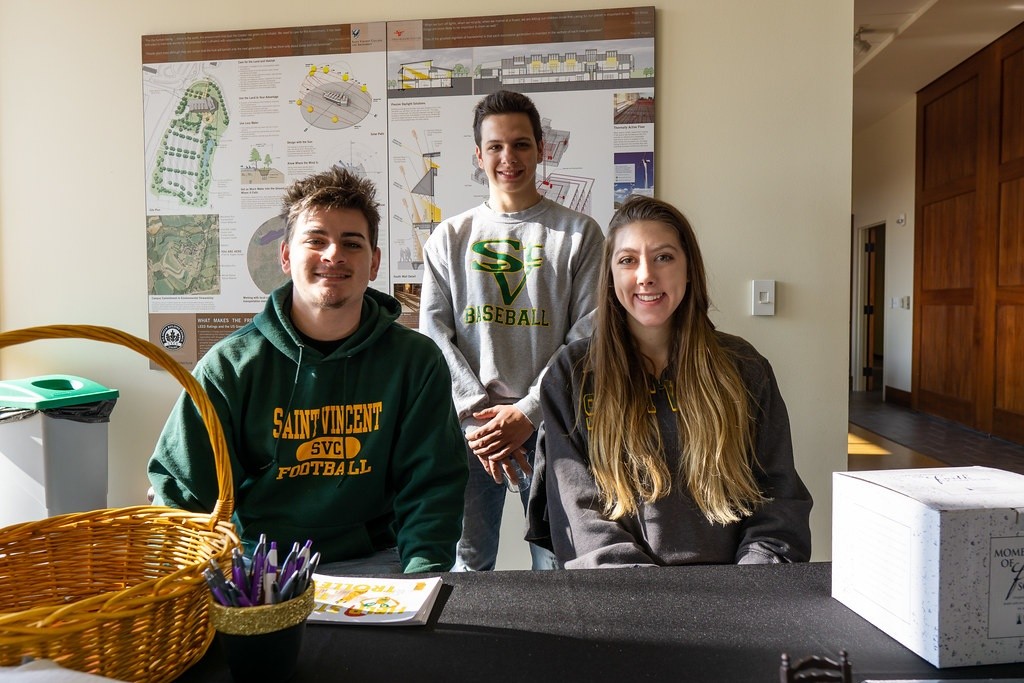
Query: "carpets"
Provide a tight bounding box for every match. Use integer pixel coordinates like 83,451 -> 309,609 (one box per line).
849,390 -> 1024,476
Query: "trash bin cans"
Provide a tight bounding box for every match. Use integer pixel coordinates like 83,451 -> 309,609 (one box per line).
0,374 -> 121,534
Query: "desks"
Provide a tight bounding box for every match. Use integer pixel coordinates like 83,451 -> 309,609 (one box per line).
172,561 -> 1024,683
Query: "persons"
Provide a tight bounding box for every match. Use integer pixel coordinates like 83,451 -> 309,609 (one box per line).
417,92 -> 606,572
146,167 -> 470,574
540,198 -> 813,569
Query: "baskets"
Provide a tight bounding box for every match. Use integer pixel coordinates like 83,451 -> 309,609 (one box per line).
208,576 -> 316,636
1,322 -> 243,682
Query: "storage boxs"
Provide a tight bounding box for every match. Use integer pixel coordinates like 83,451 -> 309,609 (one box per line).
831,465 -> 1024,669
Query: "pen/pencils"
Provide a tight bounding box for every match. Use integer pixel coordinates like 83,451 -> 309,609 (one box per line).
204,533 -> 322,607
